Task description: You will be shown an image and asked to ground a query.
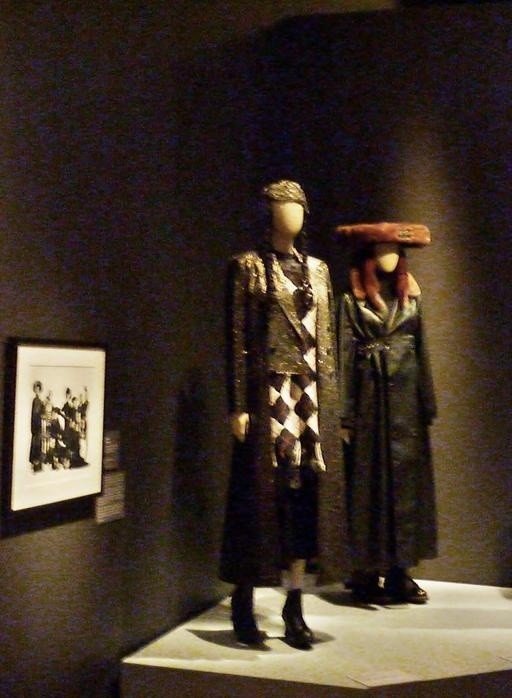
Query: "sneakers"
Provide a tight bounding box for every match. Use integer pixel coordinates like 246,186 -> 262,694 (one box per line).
282,604 -> 316,646
350,582 -> 397,605
229,593 -> 260,647
384,574 -> 428,604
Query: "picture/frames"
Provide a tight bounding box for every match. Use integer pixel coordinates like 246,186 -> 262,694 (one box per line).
4,338 -> 110,514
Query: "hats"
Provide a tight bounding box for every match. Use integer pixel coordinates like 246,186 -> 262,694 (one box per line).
261,181 -> 315,315
333,221 -> 433,248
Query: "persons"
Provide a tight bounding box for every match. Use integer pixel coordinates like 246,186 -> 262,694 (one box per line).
28,380 -> 88,472
221,180 -> 336,646
337,221 -> 437,607
